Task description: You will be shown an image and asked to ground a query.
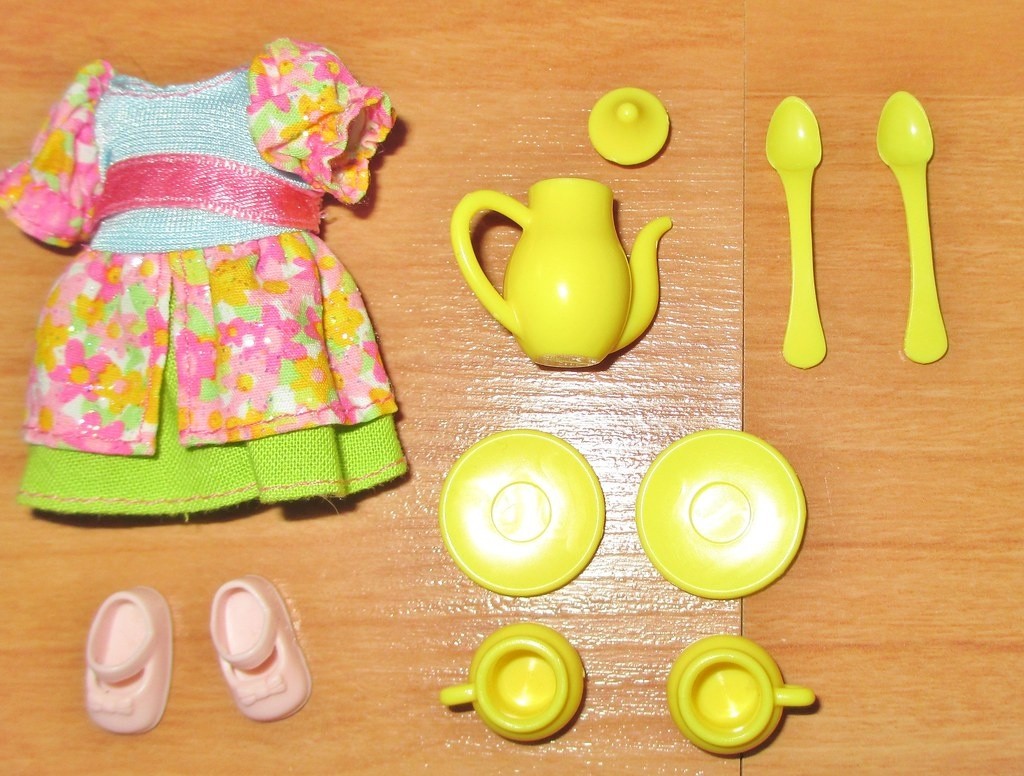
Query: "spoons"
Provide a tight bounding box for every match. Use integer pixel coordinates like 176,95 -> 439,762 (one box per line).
877,91 -> 948,364
763,94 -> 830,370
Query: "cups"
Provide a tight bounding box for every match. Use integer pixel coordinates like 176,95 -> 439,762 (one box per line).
666,634 -> 815,758
437,625 -> 591,744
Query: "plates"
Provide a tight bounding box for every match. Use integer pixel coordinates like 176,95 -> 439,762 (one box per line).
436,429 -> 608,601
636,430 -> 807,603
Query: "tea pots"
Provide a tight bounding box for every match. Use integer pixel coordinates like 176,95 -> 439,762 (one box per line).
453,88 -> 674,368
